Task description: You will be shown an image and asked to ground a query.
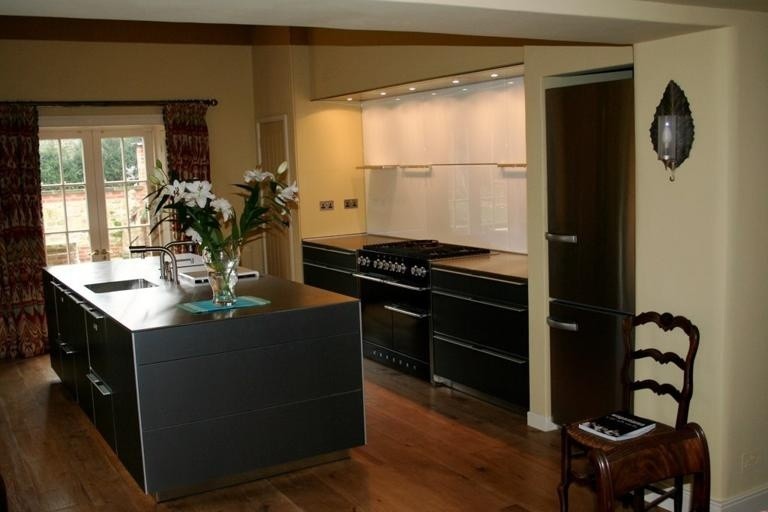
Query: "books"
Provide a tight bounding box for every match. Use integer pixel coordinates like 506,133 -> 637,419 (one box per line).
580,410 -> 655,443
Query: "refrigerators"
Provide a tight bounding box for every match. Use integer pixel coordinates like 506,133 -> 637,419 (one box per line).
544,78 -> 636,433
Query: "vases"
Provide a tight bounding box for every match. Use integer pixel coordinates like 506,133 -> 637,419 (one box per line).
202,244 -> 241,306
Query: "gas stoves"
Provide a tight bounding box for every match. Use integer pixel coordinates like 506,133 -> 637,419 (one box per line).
357,239 -> 492,283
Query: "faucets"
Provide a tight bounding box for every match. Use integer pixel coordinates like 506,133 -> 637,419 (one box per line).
129,245 -> 180,285
159,240 -> 195,279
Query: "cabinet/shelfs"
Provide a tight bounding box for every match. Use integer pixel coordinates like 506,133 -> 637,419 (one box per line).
40,252 -> 367,503
301,241 -> 357,299
429,253 -> 529,413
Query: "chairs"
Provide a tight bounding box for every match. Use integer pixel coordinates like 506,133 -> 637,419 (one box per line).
557,310 -> 701,512
589,420 -> 712,510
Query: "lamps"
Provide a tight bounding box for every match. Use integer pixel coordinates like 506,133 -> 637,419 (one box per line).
649,80 -> 696,181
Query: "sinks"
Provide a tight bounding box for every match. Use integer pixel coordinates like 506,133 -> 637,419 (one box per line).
158,262 -> 209,274
83,278 -> 159,294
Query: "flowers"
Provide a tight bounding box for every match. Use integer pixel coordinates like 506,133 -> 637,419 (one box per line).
130,158 -> 301,299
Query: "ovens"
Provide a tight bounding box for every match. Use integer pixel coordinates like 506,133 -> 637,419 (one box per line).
352,268 -> 432,383
